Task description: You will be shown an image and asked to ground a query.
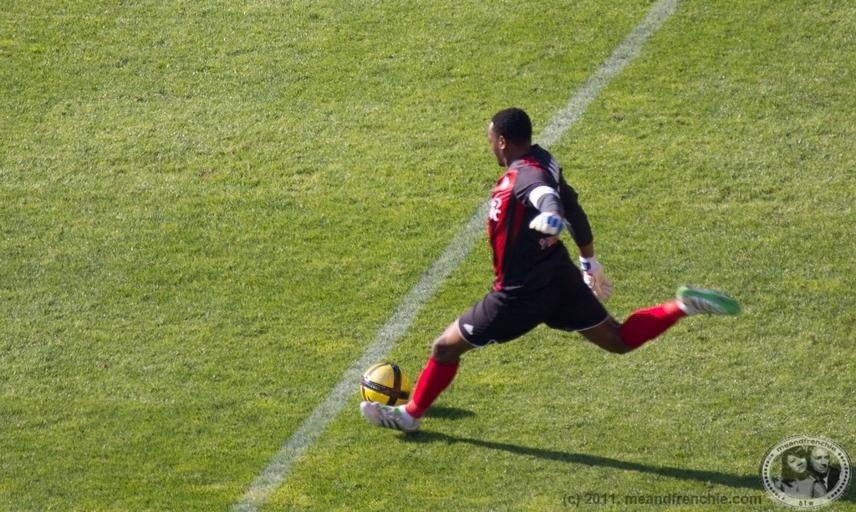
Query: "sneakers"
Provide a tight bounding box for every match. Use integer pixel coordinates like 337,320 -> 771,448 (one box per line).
675,286 -> 742,316
358,400 -> 421,434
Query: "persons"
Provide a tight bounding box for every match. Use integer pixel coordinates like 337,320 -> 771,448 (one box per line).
772,443 -> 825,499
804,443 -> 840,491
356,106 -> 742,434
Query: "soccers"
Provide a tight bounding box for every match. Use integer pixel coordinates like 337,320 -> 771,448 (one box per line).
360,362 -> 411,406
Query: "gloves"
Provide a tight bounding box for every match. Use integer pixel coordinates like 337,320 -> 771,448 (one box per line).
528,212 -> 572,238
578,254 -> 614,304
